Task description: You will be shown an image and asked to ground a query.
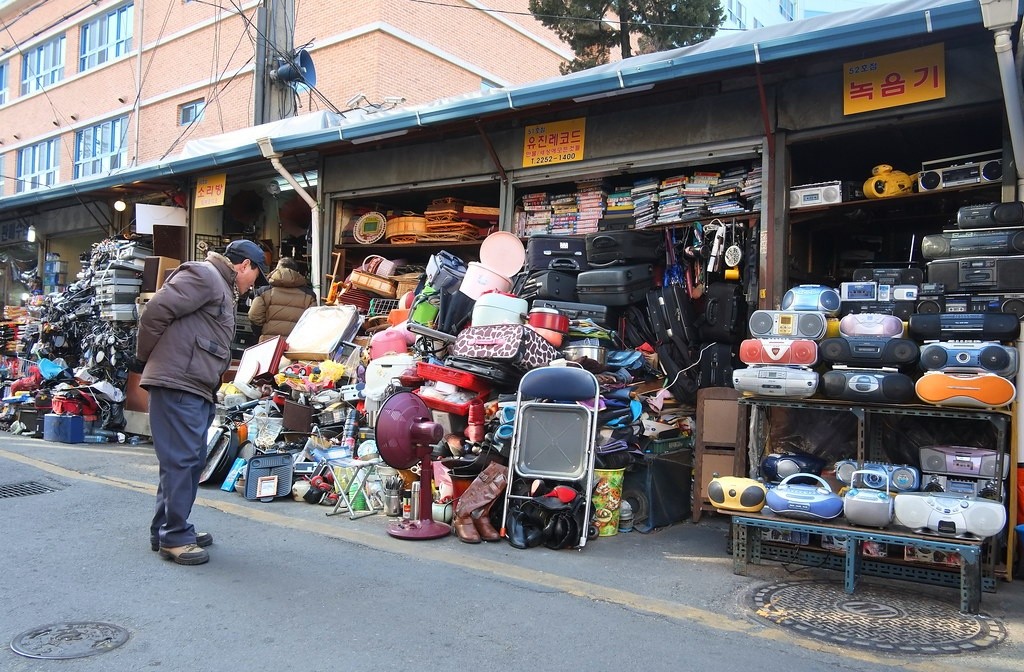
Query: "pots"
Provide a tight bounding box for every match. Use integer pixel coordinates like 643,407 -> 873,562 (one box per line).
471,293 -> 528,326
520,303 -> 605,369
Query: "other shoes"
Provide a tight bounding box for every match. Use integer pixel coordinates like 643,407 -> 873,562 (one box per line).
451,402 -> 586,550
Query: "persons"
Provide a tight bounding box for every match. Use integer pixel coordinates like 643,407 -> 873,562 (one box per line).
248,258 -> 319,345
129,240 -> 267,567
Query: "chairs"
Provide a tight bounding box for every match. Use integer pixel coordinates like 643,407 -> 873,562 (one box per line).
499,366 -> 600,550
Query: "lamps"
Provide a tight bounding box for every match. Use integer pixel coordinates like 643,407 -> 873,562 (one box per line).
114,195 -> 127,212
266,180 -> 283,258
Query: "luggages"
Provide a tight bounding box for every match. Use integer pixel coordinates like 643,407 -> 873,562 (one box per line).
452,229 -> 741,387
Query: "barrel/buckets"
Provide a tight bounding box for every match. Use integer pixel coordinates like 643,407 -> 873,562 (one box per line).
588,467 -> 627,536
459,261 -> 512,301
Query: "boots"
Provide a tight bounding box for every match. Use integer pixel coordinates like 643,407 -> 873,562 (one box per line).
452,461 -> 508,544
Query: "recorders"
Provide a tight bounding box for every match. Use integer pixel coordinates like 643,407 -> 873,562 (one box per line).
707,445 -> 1011,540
733,147 -> 1024,408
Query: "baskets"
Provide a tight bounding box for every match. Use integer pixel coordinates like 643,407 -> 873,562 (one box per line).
338,255 -> 421,346
385,196 -> 480,247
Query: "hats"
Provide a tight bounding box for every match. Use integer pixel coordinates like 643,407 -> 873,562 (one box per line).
223,239 -> 271,286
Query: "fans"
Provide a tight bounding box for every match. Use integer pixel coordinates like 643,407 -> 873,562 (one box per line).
374,392 -> 453,540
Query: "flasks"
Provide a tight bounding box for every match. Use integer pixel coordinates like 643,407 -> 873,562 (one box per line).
411,481 -> 421,521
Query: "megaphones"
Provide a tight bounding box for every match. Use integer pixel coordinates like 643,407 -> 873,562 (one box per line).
270,50 -> 317,89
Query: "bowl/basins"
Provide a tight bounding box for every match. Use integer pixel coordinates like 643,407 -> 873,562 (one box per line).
234,481 -> 245,494
432,502 -> 453,523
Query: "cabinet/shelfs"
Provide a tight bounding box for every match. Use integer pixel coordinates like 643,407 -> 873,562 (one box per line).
0,178 -> 1023,616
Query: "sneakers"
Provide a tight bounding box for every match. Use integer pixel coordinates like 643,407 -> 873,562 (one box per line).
150,531 -> 213,551
158,543 -> 209,565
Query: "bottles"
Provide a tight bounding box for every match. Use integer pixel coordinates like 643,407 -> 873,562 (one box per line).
403,489 -> 412,520
83,435 -> 109,443
91,428 -> 117,437
385,481 -> 400,516
349,483 -> 366,511
292,480 -> 312,502
370,480 -> 385,510
130,437 -> 139,445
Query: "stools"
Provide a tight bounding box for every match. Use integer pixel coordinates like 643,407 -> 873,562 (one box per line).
325,457 -> 382,521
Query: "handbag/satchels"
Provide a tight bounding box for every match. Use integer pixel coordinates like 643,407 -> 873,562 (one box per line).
425,250 -> 476,337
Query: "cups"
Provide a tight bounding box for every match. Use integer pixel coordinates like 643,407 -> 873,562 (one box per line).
365,411 -> 377,428
